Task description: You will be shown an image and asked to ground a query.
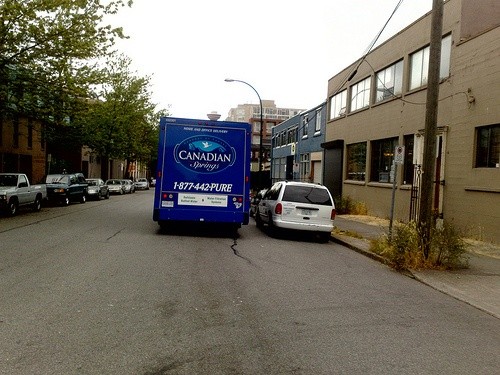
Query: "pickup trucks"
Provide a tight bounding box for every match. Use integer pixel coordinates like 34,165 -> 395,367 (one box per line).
0,173 -> 47,217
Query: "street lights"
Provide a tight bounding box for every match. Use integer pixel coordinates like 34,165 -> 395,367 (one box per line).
225,79 -> 263,190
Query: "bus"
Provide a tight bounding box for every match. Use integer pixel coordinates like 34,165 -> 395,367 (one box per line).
153,111 -> 251,233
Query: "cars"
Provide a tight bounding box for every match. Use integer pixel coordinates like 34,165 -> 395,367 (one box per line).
85,179 -> 109,201
105,178 -> 150,195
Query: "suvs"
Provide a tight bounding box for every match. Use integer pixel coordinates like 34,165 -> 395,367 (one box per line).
252,179 -> 336,244
37,171 -> 88,206
